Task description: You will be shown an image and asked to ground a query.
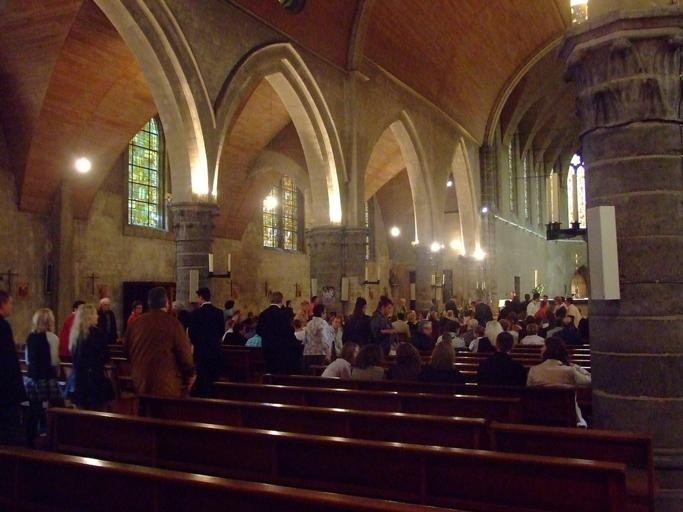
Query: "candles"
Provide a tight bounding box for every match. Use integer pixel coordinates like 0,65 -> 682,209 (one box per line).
377,265 -> 380,280
365,266 -> 368,281
209,252 -> 214,273
227,253 -> 231,272
432,275 -> 436,286
442,275 -> 445,285
476,280 -> 486,290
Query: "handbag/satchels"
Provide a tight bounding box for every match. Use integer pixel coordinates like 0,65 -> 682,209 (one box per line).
73,370 -> 115,408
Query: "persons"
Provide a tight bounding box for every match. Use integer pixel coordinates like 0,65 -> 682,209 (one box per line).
0,286 -> 591,450
